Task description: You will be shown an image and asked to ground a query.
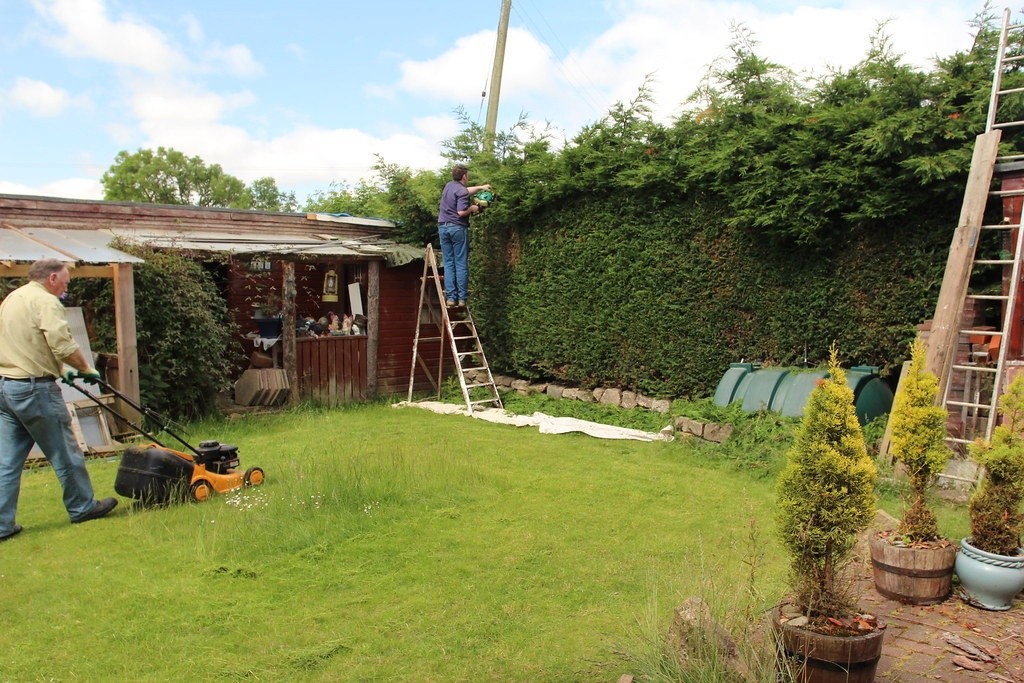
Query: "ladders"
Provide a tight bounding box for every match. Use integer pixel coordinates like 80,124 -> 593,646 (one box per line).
926,6 -> 1024,493
408,241 -> 504,415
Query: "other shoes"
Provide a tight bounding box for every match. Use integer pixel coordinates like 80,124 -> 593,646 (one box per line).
458,299 -> 466,306
446,299 -> 457,306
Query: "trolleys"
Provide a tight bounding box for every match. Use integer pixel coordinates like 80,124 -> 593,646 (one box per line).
62,373 -> 265,505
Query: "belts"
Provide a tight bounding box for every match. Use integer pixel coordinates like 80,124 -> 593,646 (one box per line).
0,376 -> 54,381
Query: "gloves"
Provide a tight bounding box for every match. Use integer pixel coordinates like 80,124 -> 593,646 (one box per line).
59,366 -> 74,386
77,364 -> 101,385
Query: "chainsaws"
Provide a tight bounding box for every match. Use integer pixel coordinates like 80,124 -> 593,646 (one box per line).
471,180 -> 502,216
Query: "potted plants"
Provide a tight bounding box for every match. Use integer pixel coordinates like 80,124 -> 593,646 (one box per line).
252,295 -> 283,338
867,329 -> 957,604
954,368 -> 1024,610
775,338 -> 888,682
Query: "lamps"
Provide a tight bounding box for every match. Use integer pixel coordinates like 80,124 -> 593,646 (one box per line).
322,261 -> 339,302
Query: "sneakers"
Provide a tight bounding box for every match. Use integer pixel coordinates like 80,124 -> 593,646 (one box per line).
0,525 -> 21,539
73,497 -> 118,523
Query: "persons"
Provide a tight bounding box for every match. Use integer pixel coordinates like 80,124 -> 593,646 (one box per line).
438,166 -> 492,309
0,260 -> 117,540
